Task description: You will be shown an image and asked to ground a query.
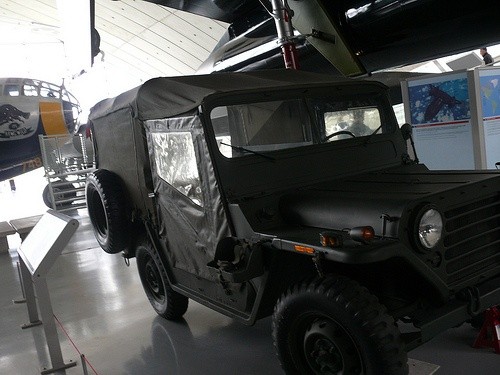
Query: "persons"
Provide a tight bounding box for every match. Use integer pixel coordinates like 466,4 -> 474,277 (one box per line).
479,47 -> 494,66
337,108 -> 377,138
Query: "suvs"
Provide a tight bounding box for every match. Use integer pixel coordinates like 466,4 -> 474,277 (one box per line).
85,69 -> 500,375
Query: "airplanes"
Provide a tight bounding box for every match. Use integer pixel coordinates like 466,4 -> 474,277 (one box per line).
0,78 -> 83,210
